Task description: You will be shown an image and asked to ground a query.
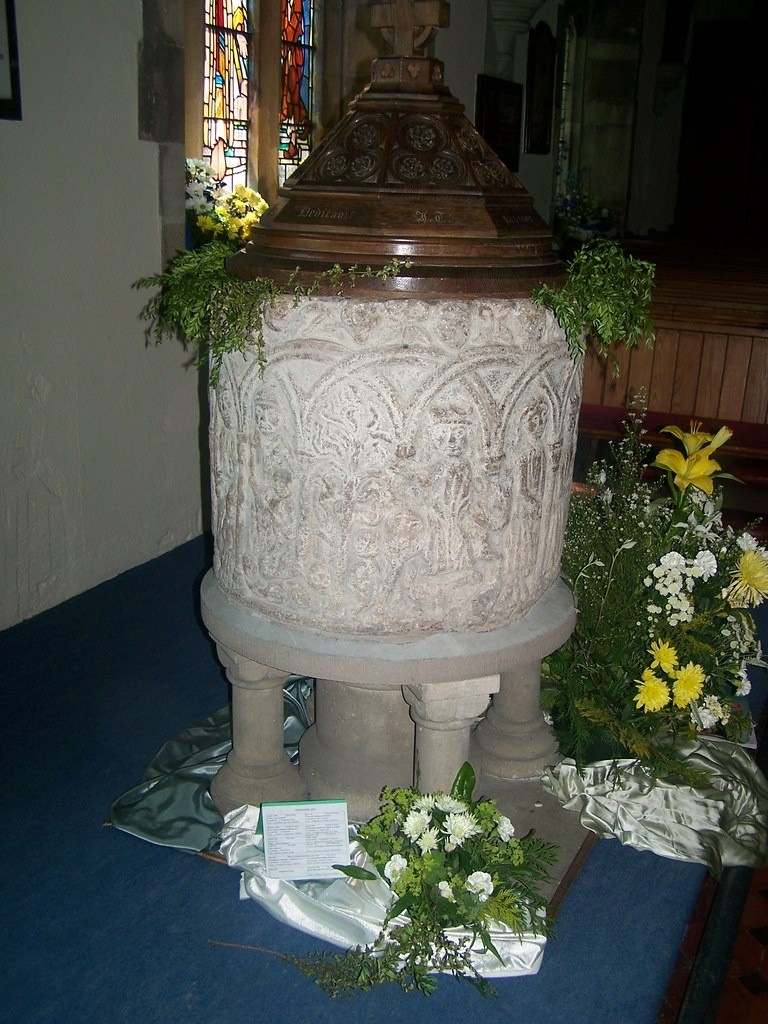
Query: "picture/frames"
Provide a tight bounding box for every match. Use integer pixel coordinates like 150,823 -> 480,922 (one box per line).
478,74 -> 522,173
0,0 -> 23,121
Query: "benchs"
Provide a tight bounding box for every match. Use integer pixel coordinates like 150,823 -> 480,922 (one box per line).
580,227 -> 767,545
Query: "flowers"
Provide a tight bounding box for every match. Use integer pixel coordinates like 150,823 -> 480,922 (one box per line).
183,158 -> 269,249
537,386 -> 768,790
208,763 -> 561,1000
551,119 -> 623,241
